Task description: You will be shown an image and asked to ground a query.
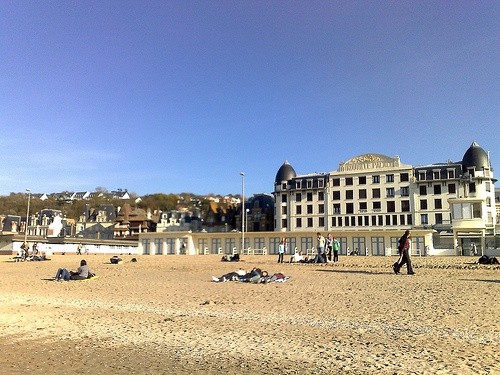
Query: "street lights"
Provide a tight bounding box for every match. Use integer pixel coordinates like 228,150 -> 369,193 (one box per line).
246,209 -> 249,232
22,189 -> 31,258
240,172 -> 245,261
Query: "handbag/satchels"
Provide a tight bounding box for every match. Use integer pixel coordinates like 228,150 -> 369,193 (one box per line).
394,262 -> 399,268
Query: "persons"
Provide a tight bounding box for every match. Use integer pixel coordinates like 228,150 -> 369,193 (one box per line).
220,253 -> 240,262
211,267 -> 286,284
277,240 -> 284,263
53,259 -> 95,282
76,241 -> 83,256
290,232 -> 340,263
478,254 -> 500,265
19,242 -> 47,262
391,231 -> 416,275
180,242 -> 186,254
350,248 -> 360,256
470,243 -> 478,256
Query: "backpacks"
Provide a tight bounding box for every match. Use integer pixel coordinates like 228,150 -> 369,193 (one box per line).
235,254 -> 239,259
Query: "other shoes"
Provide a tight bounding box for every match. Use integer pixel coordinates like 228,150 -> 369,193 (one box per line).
393,268 -> 397,274
408,272 -> 416,275
